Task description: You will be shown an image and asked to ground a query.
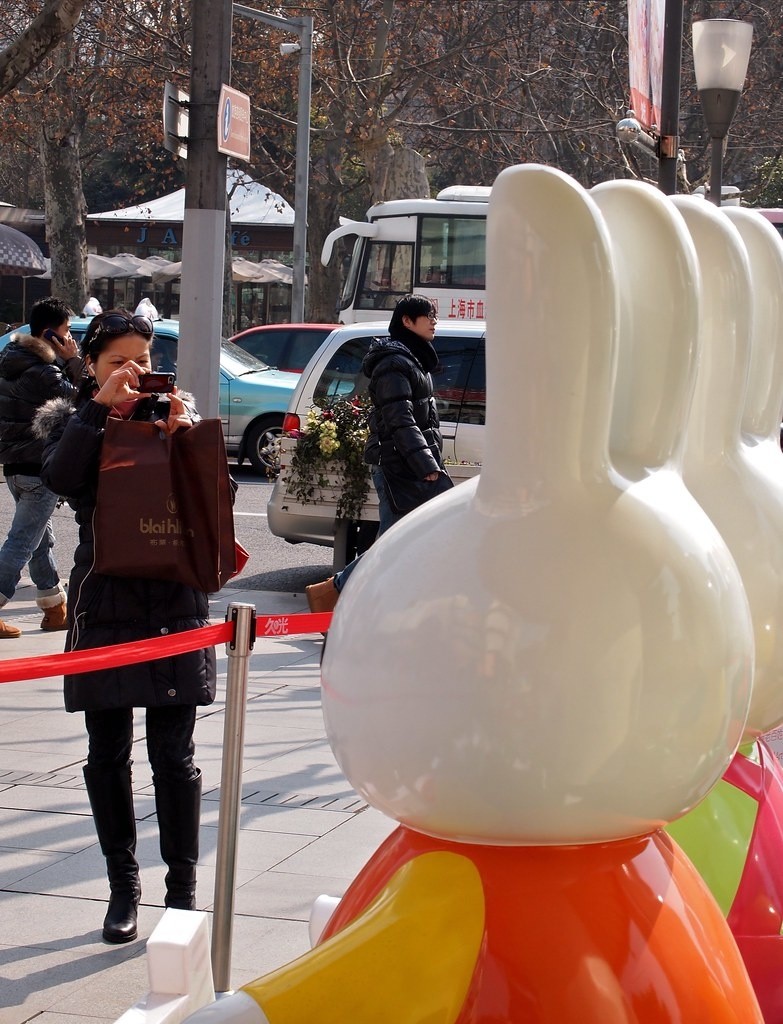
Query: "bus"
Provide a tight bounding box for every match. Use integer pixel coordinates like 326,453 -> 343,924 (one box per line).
320,181 -> 783,322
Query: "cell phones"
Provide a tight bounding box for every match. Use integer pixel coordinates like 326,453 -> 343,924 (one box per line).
135,372 -> 175,393
43,329 -> 65,346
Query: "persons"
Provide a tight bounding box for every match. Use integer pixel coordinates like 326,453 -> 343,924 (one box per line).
31,303 -> 239,944
0,299 -> 91,640
306,292 -> 457,637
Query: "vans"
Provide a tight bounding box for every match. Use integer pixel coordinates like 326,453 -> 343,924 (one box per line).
267,319 -> 488,553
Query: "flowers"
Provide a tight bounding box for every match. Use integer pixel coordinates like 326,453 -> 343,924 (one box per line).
263,391 -> 372,537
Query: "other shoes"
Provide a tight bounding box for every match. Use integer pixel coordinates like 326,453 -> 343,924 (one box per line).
304,574 -> 341,637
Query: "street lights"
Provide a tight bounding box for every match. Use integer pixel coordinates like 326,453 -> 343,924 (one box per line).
692,18 -> 754,207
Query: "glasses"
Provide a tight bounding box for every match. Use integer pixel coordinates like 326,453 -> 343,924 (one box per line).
88,314 -> 153,354
425,311 -> 440,324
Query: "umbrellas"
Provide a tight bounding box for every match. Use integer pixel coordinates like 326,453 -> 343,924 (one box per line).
0,222 -> 47,274
21,250 -> 310,286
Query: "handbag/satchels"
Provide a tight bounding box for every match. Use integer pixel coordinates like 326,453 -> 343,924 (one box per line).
364,437 -> 406,465
90,415 -> 236,594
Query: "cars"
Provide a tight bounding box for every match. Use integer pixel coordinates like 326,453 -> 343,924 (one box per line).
0,298 -> 358,484
228,322 -> 345,374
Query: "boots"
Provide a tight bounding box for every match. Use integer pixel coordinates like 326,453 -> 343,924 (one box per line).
35,590 -> 71,631
82,763 -> 142,944
0,592 -> 22,638
152,766 -> 202,911
